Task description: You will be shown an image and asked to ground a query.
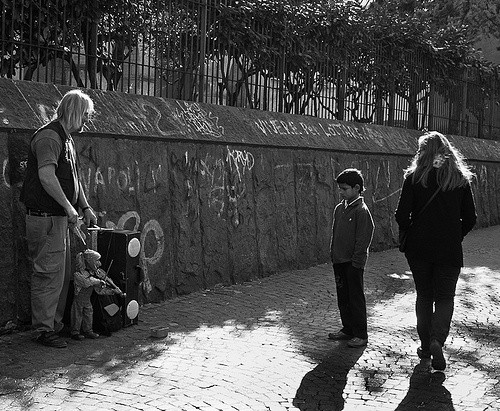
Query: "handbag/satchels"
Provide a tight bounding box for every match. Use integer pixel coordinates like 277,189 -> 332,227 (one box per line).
399,224 -> 411,253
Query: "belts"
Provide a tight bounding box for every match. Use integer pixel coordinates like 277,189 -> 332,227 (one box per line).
27,208 -> 55,217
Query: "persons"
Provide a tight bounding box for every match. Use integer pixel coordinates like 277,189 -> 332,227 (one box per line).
329,168 -> 375,347
395,132 -> 478,370
19,89 -> 97,348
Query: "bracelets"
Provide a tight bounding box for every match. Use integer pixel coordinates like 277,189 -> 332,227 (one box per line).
82,206 -> 89,213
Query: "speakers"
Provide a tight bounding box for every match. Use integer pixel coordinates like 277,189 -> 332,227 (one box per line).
98,229 -> 141,327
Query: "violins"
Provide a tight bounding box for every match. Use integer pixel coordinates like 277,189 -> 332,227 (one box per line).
95,268 -> 127,299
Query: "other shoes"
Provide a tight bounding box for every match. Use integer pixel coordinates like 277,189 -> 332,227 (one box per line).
32,331 -> 67,347
328,331 -> 353,340
347,337 -> 368,348
430,340 -> 446,370
417,347 -> 431,359
56,331 -> 71,340
